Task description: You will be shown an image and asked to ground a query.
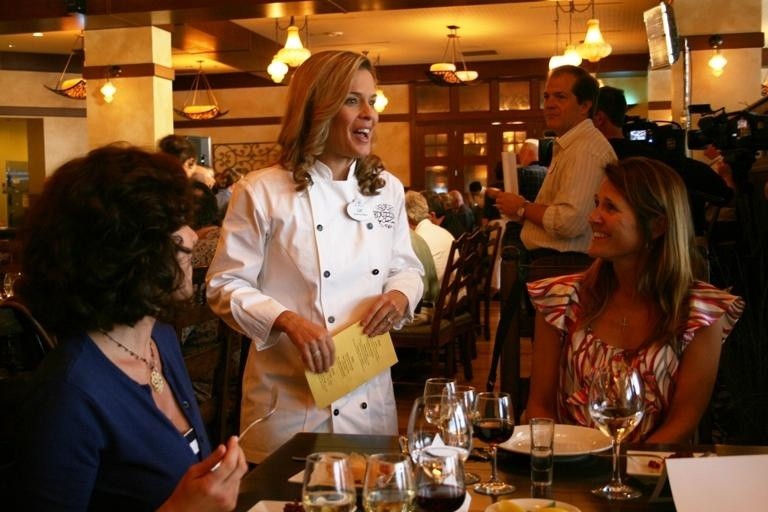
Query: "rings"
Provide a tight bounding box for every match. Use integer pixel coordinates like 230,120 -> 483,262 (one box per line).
386,317 -> 392,323
313,351 -> 320,357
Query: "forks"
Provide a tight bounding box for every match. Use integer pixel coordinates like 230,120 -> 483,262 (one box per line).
209,385 -> 280,472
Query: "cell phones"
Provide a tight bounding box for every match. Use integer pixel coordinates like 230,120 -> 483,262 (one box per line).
630,129 -> 647,141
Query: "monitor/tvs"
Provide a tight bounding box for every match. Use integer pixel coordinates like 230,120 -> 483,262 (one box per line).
643,1 -> 680,70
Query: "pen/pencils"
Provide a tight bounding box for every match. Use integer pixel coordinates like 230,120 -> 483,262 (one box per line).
493,200 -> 530,206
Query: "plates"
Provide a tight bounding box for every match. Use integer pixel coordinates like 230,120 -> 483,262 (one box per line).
498,423 -> 613,461
625,449 -> 720,485
245,499 -> 357,512
288,459 -> 394,490
485,498 -> 581,512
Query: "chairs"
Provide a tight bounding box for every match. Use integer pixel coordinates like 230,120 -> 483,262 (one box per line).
500,247 -> 593,425
465,222 -> 502,359
167,265 -> 244,440
0,301 -> 57,378
391,229 -> 486,390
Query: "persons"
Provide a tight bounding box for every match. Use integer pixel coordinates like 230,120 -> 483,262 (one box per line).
654,124 -> 729,291
446,190 -> 476,234
425,195 -> 472,278
160,137 -> 216,226
469,183 -> 486,222
702,139 -> 737,227
405,227 -> 440,307
724,113 -> 757,187
16,144 -> 248,512
205,53 -> 424,478
524,158 -> 742,445
592,87 -> 642,165
484,70 -> 622,465
403,191 -> 466,377
189,185 -> 219,243
495,144 -> 550,263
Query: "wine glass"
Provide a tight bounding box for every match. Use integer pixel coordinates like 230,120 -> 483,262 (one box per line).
4,272 -> 21,298
588,366 -> 644,500
301,451 -> 357,512
408,376 -> 518,512
362,453 -> 412,512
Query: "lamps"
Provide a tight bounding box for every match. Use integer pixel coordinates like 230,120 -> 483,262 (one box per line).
174,60 -> 230,122
267,16 -> 312,85
429,26 -> 479,85
708,35 -> 727,76
40,30 -> 86,98
100,70 -> 116,105
549,0 -> 612,71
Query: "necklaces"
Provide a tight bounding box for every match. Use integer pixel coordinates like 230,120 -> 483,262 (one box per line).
611,298 -> 647,334
98,330 -> 163,396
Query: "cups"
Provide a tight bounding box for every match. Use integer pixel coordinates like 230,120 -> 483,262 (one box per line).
530,418 -> 556,487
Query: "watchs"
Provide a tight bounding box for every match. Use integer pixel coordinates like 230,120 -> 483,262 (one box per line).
516,200 -> 529,220
